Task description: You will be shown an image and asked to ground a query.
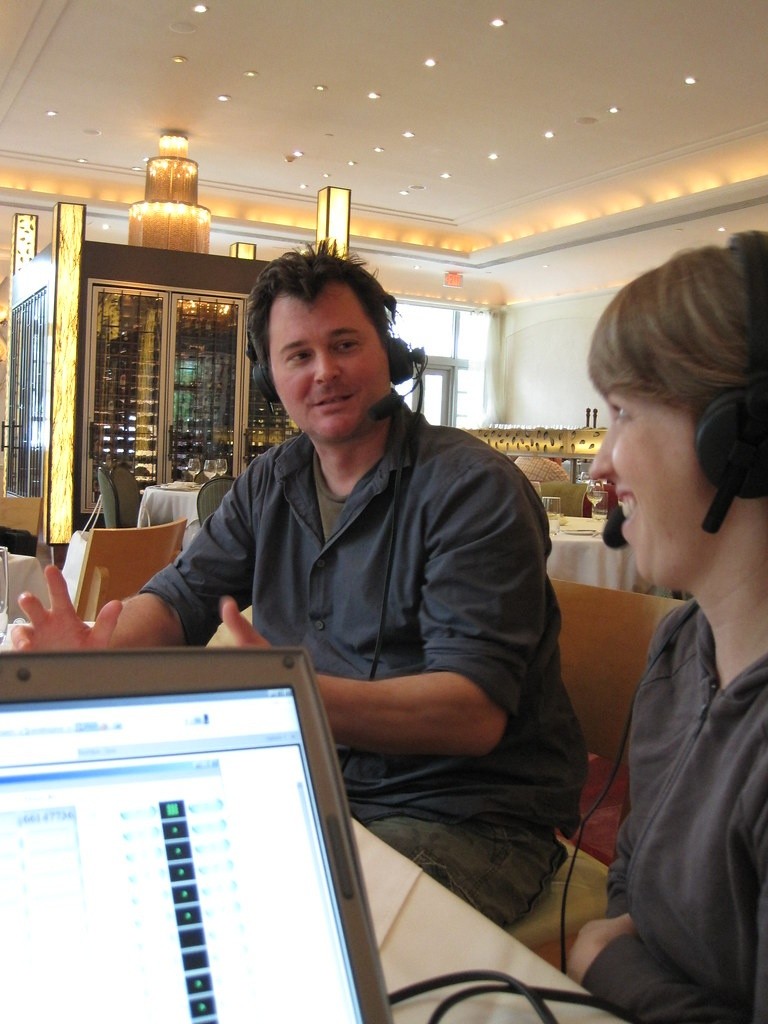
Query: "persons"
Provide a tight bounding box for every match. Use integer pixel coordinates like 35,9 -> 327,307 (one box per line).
8,246 -> 588,933
564,230 -> 768,1024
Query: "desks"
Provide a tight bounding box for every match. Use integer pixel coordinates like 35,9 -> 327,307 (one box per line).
0,552 -> 51,618
351,817 -> 627,1024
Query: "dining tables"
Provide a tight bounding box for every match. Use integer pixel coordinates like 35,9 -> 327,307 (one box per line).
546,515 -> 672,599
136,483 -> 203,549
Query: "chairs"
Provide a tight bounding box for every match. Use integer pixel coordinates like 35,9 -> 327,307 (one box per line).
97,462 -> 140,528
542,481 -> 593,519
196,476 -> 235,528
75,516 -> 188,622
505,579 -> 686,955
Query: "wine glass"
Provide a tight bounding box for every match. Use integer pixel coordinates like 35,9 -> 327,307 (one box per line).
592,491 -> 609,538
204,459 -> 217,480
216,459 -> 227,476
188,458 -> 200,482
586,479 -> 604,522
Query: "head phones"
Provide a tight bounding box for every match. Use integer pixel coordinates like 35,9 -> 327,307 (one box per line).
602,229 -> 768,549
245,258 -> 428,423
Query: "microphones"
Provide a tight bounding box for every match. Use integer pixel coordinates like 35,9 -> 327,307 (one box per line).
603,505 -> 628,550
367,362 -> 424,422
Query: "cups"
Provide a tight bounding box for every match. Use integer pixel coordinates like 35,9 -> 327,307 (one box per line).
530,481 -> 541,501
541,496 -> 561,536
0,545 -> 9,644
581,471 -> 592,484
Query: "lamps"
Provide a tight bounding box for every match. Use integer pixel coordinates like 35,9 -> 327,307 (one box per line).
127,133 -> 211,253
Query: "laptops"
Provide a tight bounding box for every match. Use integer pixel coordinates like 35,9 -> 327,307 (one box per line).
0,646 -> 394,1024
